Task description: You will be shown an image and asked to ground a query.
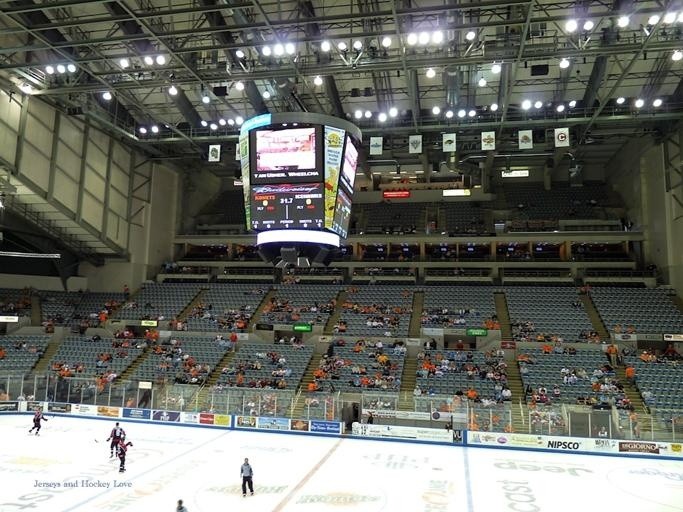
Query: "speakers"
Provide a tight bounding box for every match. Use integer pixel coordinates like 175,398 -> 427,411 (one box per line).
364,87 -> 373,96
213,86 -> 229,96
67,107 -> 82,116
350,88 -> 360,97
531,64 -> 549,76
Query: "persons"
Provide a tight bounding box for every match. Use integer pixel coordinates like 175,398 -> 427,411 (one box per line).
239,457 -> 254,497
175,499 -> 189,511
106,422 -> 125,458
117,438 -> 127,473
0,240 -> 682,440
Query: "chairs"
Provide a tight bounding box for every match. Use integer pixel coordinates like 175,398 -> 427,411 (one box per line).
191,178 -> 632,233
1,280 -> 683,439
